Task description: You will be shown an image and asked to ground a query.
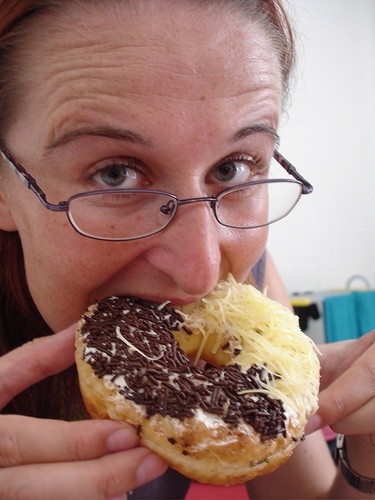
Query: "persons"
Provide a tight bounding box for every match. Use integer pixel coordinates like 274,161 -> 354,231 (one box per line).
0,0 -> 374,500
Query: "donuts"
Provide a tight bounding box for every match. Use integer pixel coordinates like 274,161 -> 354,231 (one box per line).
74,271 -> 321,486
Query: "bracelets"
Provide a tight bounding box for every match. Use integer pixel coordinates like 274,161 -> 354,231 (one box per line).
333,434 -> 374,493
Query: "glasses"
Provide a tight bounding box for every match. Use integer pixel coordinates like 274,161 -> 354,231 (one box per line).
0,143 -> 313,239
